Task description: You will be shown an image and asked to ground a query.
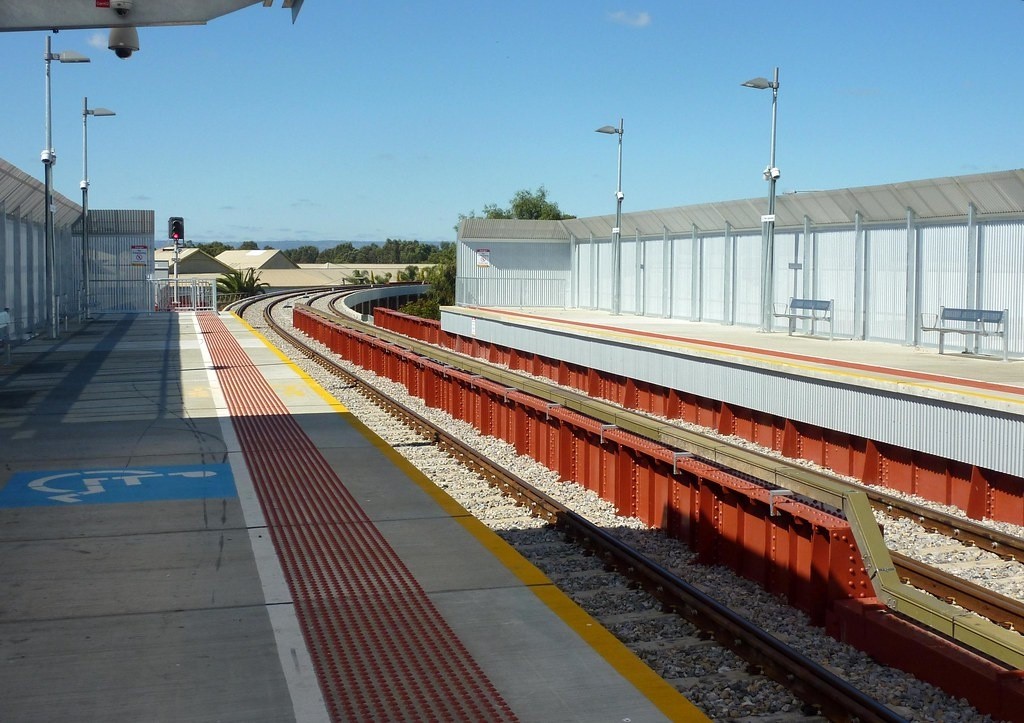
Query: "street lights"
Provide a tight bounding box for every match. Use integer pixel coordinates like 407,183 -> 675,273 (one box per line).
595,126 -> 624,316
79,106 -> 117,322
41,54 -> 92,341
739,78 -> 781,334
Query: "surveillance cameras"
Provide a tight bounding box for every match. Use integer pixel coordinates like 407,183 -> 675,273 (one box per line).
109,0 -> 134,16
107,26 -> 140,59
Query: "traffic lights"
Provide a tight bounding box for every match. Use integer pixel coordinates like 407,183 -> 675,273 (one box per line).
168,217 -> 185,239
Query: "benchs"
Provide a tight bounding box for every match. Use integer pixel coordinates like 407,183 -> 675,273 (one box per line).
921,304 -> 1008,361
773,297 -> 835,336
